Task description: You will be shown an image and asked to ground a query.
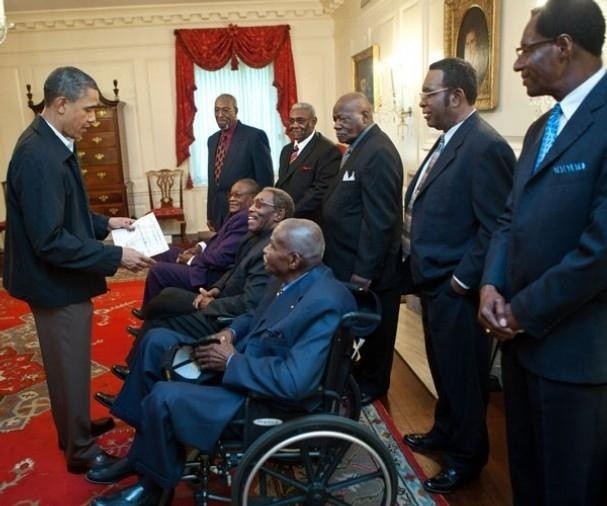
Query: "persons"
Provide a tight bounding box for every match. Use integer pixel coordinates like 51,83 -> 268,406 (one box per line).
94,186 -> 296,404
274,102 -> 341,218
475,0 -> 607,506
127,176 -> 260,335
460,25 -> 489,87
83,217 -> 358,505
2,66 -> 157,474
207,94 -> 274,232
360,76 -> 371,101
318,92 -> 401,407
400,57 -> 518,494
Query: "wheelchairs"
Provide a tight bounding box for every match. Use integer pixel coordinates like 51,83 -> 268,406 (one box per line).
182,288 -> 399,506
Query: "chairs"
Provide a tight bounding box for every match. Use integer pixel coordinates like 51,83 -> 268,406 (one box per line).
146,169 -> 189,244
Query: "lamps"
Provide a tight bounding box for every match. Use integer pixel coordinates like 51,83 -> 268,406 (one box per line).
397,106 -> 412,141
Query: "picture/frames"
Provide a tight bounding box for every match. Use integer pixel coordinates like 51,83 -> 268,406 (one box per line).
351,43 -> 382,113
442,0 -> 500,111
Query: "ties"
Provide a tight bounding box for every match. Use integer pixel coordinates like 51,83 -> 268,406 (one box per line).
532,103 -> 562,174
214,134 -> 229,183
289,145 -> 299,164
402,134 -> 444,261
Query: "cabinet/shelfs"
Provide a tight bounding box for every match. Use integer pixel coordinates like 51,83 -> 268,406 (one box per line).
27,80 -> 137,219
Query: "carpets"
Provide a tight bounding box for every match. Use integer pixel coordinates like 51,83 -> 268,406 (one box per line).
1,279 -> 457,506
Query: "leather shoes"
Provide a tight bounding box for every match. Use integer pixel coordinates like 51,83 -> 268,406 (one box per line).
59,308 -> 146,506
424,468 -> 463,495
403,432 -> 438,457
342,389 -> 373,408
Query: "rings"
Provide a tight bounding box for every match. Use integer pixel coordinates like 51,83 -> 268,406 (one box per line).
485,328 -> 491,335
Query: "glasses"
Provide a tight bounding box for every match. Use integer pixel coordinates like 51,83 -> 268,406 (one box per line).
247,198 -> 281,210
515,36 -> 556,57
419,86 -> 451,102
